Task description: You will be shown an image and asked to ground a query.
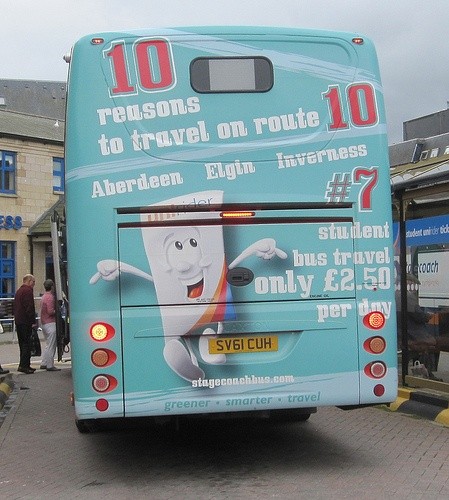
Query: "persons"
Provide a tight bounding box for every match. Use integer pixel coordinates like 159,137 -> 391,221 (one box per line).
40,279 -> 61,371
12,274 -> 36,374
60,289 -> 69,356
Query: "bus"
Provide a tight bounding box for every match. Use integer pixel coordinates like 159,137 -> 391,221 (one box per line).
50,24 -> 398,431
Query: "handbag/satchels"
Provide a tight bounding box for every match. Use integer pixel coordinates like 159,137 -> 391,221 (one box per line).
411,361 -> 428,377
31,328 -> 41,356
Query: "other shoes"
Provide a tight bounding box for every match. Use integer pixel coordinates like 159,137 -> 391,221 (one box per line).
40,366 -> 47,368
47,367 -> 61,371
0,369 -> 9,374
18,367 -> 36,372
20,367 -> 34,374
